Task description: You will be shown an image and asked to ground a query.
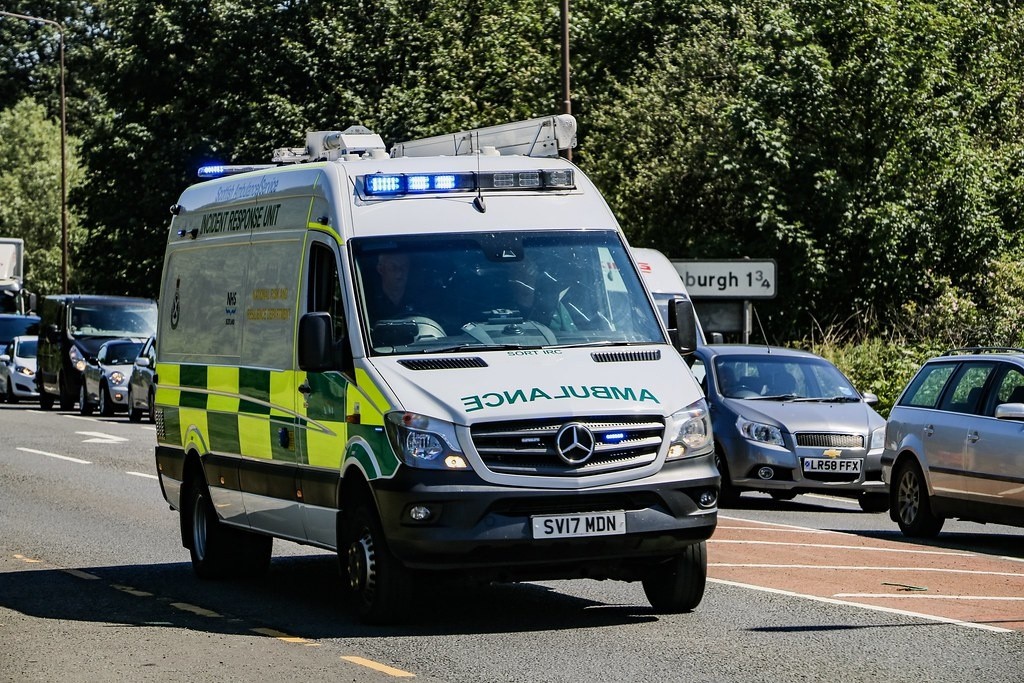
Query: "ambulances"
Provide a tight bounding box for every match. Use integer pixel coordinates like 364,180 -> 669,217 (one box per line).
153,113 -> 718,614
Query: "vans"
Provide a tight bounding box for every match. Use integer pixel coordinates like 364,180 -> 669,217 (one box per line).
587,241 -> 715,397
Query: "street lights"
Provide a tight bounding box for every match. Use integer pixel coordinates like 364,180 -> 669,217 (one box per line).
558,0 -> 579,166
0,9 -> 68,298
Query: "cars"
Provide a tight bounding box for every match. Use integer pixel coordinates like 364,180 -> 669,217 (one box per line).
696,342 -> 890,513
125,333 -> 174,421
2,293 -> 152,415
886,355 -> 1024,537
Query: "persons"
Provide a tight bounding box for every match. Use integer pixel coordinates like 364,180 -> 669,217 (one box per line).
367,250 -> 411,326
718,367 -> 736,388
501,256 -> 577,332
763,372 -> 796,396
